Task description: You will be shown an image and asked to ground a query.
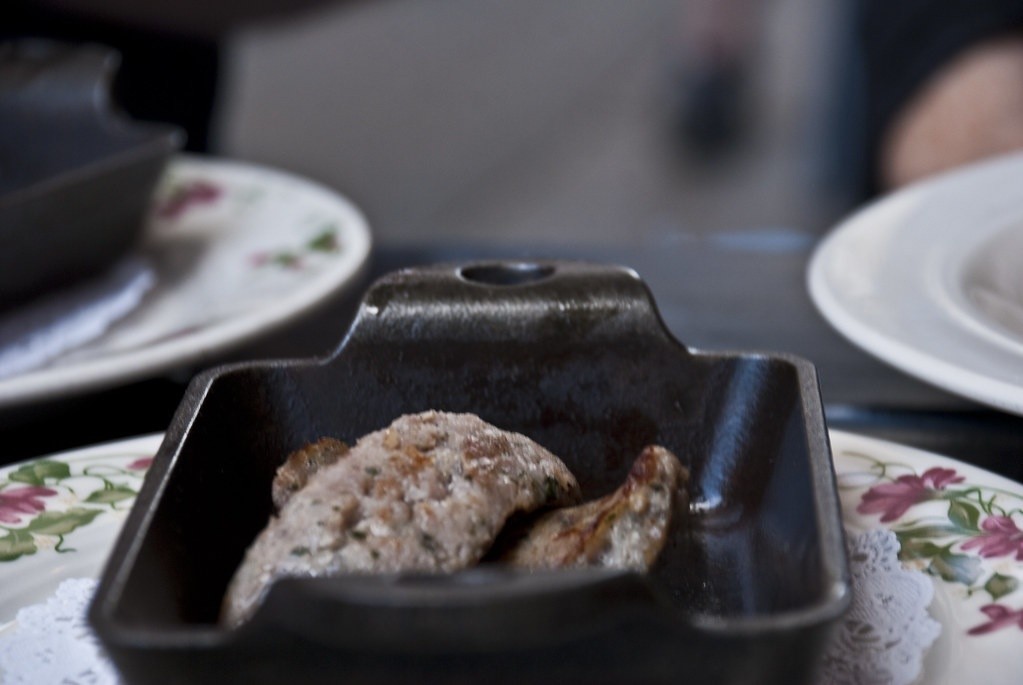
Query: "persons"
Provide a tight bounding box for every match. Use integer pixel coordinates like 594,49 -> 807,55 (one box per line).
853,1 -> 1022,206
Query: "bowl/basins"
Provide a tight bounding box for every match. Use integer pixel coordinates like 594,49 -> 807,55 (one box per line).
88,256 -> 852,685
1,49 -> 179,318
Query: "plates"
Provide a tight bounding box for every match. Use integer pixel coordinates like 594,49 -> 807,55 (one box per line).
4,426 -> 1022,683
803,150 -> 1023,431
0,159 -> 371,409
